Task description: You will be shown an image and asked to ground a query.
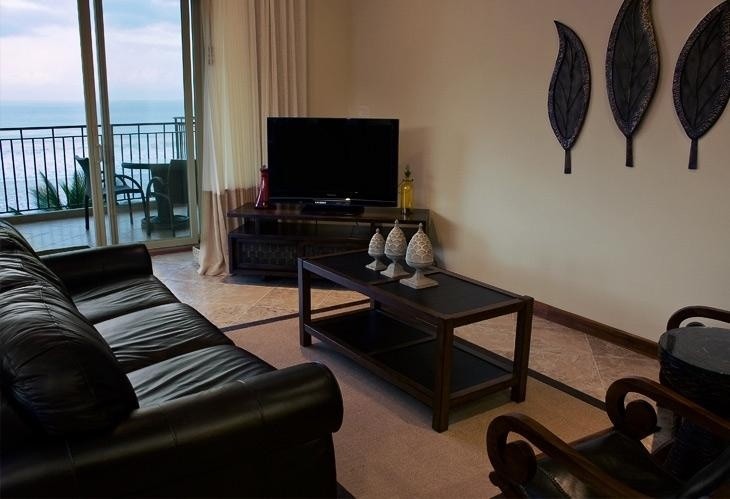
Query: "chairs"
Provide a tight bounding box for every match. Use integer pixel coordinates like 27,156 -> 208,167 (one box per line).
74,154 -> 198,238
486,376 -> 730,499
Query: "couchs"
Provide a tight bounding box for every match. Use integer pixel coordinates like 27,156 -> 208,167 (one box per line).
1,219 -> 343,499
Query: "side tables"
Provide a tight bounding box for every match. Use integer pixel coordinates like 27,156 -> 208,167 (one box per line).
651,326 -> 730,453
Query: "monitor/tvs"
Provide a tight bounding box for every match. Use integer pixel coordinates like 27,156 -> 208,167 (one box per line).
266,117 -> 401,216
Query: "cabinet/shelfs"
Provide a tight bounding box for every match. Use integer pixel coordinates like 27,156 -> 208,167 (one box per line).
227,203 -> 430,286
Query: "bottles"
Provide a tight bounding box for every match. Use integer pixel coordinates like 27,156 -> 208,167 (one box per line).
254,164 -> 269,209
400,162 -> 417,220
